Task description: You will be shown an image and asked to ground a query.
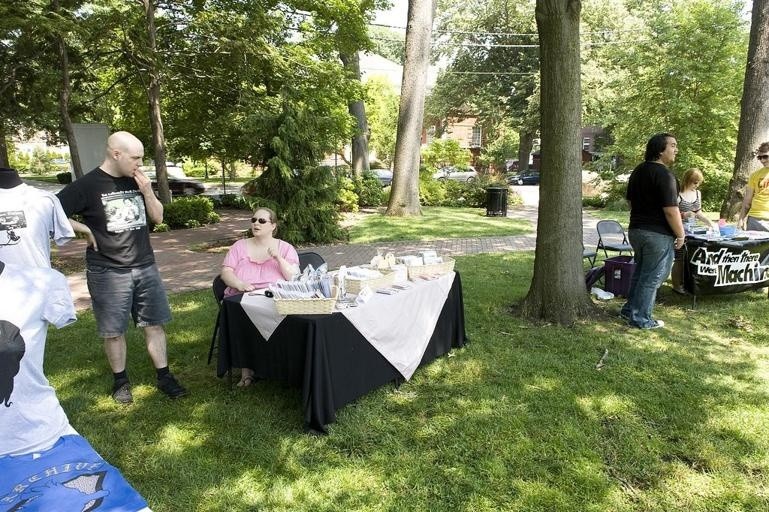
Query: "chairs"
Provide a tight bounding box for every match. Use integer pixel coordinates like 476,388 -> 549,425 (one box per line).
592,219 -> 634,266
583,242 -> 603,286
297,251 -> 324,273
207,274 -> 225,364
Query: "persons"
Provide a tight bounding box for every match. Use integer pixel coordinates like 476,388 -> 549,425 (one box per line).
619,133 -> 686,329
736,143 -> 769,294
0,259 -> 76,457
670,167 -> 714,294
0,166 -> 76,268
59,131 -> 190,405
219,208 -> 300,387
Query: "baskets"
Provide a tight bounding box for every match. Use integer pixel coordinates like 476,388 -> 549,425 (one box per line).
273,285 -> 339,315
327,264 -> 395,295
394,253 -> 456,281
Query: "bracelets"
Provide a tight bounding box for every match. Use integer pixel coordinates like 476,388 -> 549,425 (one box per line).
677,236 -> 685,240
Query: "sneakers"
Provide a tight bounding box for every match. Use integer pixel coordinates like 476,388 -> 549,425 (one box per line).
157,373 -> 188,399
112,375 -> 133,403
619,308 -> 655,320
627,318 -> 665,330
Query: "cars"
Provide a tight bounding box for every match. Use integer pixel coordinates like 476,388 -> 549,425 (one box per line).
164,162 -> 174,167
505,170 -> 541,186
434,163 -> 478,184
370,168 -> 393,190
505,159 -> 521,172
144,169 -> 204,198
49,158 -> 66,165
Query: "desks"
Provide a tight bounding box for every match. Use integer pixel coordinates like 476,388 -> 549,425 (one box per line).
683,224 -> 769,310
217,251 -> 469,435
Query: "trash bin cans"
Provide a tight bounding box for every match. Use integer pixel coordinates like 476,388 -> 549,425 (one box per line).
486,187 -> 508,217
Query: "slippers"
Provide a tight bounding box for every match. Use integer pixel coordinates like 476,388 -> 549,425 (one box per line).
236,375 -> 258,389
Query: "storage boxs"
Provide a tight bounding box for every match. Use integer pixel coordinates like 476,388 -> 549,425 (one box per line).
602,254 -> 636,298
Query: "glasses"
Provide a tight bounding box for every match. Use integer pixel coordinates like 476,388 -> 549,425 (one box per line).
757,155 -> 769,160
251,217 -> 273,225
